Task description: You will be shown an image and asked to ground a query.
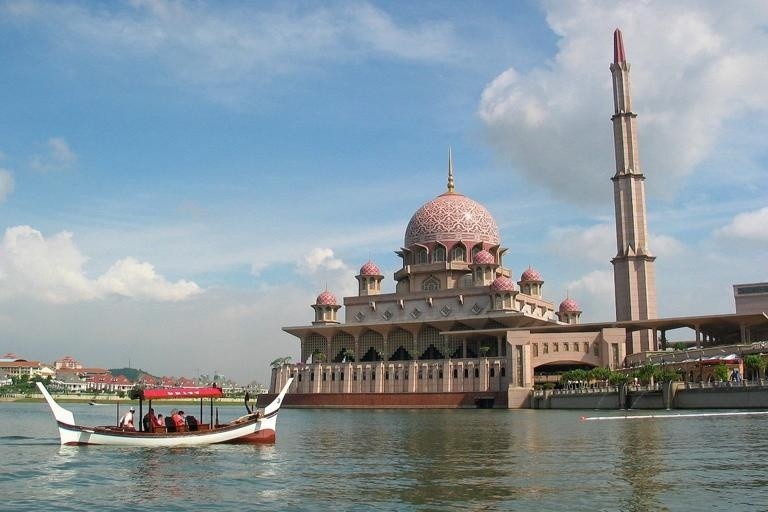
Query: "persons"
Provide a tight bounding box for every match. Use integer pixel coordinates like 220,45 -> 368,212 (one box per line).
633,377 -> 641,392
731,367 -> 741,386
118,406 -> 198,432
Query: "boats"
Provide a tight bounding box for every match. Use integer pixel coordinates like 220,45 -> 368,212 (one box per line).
35,372 -> 297,448
87,399 -> 96,406
